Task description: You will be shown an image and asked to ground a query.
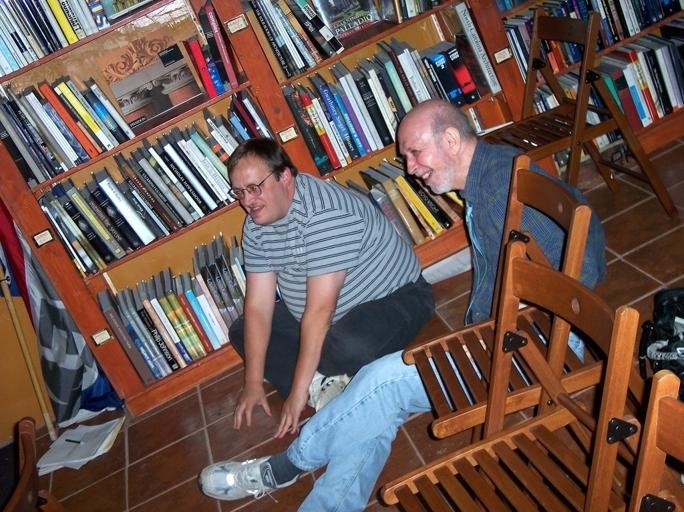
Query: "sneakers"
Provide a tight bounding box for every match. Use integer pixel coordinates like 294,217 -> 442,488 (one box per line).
197,455 -> 303,504
314,373 -> 352,413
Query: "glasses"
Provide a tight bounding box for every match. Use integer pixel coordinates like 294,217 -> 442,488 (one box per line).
227,167 -> 282,200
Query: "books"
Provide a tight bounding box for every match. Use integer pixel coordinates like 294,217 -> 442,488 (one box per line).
74,416 -> 128,459
36,422 -> 118,471
0,0 -> 683,387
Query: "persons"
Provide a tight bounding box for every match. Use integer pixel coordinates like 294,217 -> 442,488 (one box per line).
197,98 -> 608,512
224,137 -> 437,443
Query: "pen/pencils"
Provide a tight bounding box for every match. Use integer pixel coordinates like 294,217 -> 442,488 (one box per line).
64,438 -> 80,444
